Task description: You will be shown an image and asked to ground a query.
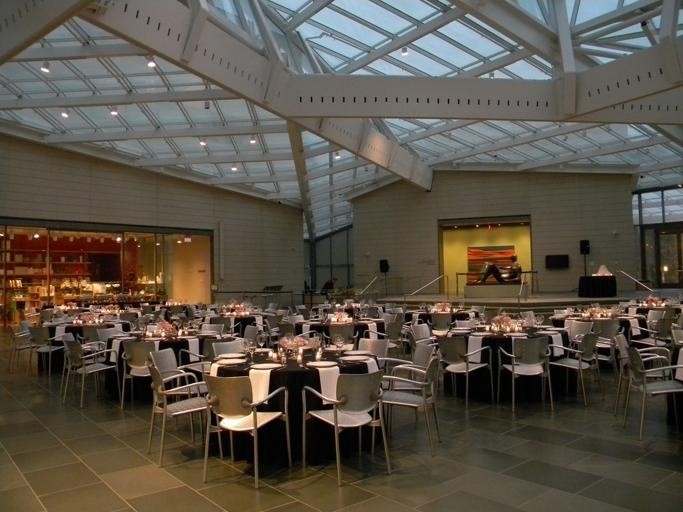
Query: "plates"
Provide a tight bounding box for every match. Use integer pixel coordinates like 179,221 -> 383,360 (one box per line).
296,318 -> 385,325
111,329 -> 217,341
217,346 -> 371,370
450,325 -> 567,336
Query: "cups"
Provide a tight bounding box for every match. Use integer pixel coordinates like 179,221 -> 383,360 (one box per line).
483,322 -> 523,332
268,348 -> 323,363
129,317 -> 202,336
144,328 -> 189,338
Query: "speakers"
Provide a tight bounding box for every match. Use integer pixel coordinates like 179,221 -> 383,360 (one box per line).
580,240 -> 590,255
380,259 -> 389,273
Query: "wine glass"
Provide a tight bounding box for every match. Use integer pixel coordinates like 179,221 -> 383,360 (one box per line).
467,313 -> 544,335
240,331 -> 345,366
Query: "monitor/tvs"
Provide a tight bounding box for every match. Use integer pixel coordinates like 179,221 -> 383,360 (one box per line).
578,276 -> 617,297
546,255 -> 569,268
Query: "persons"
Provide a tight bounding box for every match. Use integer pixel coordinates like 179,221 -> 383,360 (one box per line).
473,254 -> 522,283
321,277 -> 338,302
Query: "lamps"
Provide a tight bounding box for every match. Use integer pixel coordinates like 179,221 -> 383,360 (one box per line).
200,139 -> 207,146
401,47 -> 408,56
111,106 -> 118,116
232,164 -> 238,172
335,151 -> 340,160
40,61 -> 50,74
249,137 -> 257,145
61,110 -> 69,118
145,55 -> 157,68
